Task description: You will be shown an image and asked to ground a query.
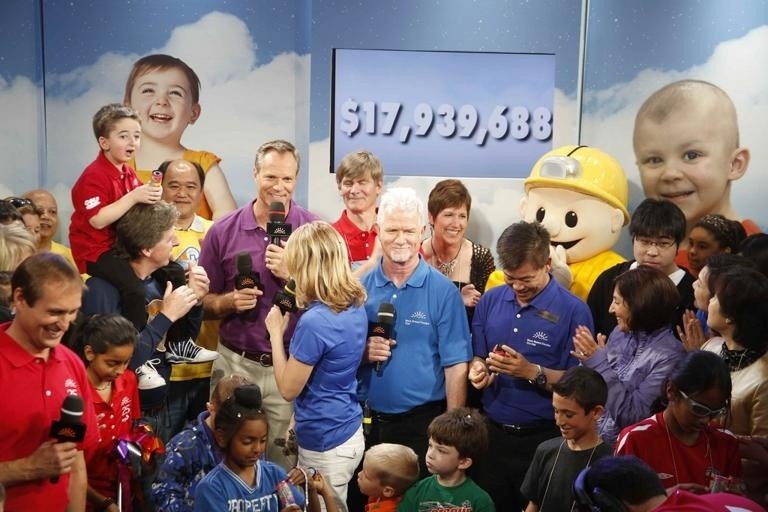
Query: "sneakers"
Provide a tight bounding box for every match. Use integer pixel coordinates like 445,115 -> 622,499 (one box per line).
165,338 -> 218,364
137,358 -> 165,390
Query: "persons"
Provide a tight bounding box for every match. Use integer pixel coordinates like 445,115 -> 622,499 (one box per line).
1,105 -> 768,512
633,79 -> 759,271
120,53 -> 240,223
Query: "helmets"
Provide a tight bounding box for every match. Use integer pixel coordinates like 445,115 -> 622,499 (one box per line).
523,146 -> 630,222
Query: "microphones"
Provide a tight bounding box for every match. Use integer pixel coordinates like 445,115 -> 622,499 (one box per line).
265,200 -> 293,249
233,251 -> 262,319
265,275 -> 297,342
48,393 -> 88,484
367,301 -> 397,375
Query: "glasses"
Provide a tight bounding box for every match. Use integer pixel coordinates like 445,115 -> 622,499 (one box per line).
10,198 -> 32,207
95,107 -> 141,132
679,392 -> 722,417
631,234 -> 677,247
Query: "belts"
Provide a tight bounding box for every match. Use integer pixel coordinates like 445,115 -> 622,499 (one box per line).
364,402 -> 430,421
479,417 -> 556,435
219,338 -> 271,366
